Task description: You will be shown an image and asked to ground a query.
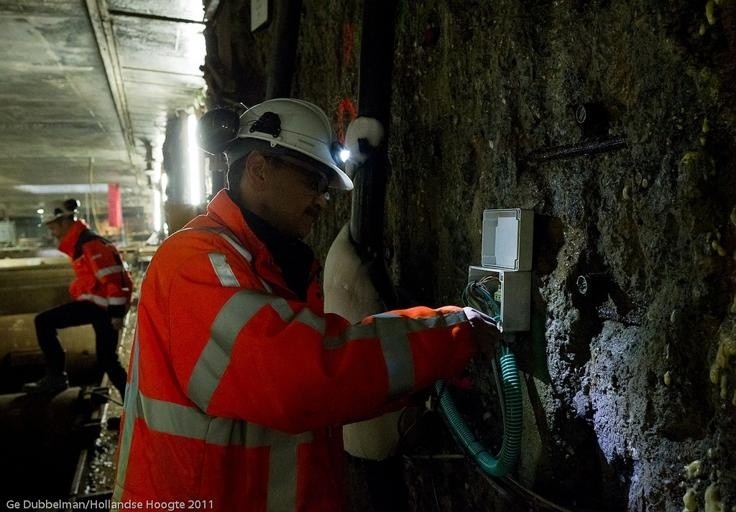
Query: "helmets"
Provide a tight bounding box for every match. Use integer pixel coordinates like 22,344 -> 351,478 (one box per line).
39,201 -> 76,224
238,99 -> 354,192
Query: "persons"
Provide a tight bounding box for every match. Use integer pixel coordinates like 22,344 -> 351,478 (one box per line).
21,199 -> 132,427
109,99 -> 495,511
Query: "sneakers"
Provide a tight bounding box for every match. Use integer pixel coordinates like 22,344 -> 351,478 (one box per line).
23,371 -> 68,392
